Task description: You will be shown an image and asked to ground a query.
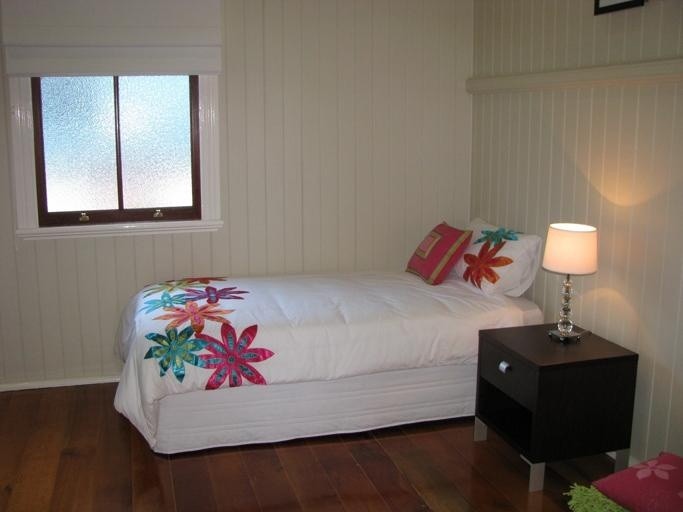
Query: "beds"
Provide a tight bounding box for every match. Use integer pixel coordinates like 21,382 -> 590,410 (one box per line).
119,270 -> 546,454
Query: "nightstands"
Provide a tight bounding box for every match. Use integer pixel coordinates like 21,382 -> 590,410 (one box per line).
476,322 -> 641,494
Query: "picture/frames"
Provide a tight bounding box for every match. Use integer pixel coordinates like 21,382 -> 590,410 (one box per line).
595,1 -> 647,15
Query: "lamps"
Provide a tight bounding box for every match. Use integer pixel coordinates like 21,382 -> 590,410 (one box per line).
541,224 -> 599,347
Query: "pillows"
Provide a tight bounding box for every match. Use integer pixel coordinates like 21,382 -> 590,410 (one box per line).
403,217 -> 544,300
591,451 -> 683,511
